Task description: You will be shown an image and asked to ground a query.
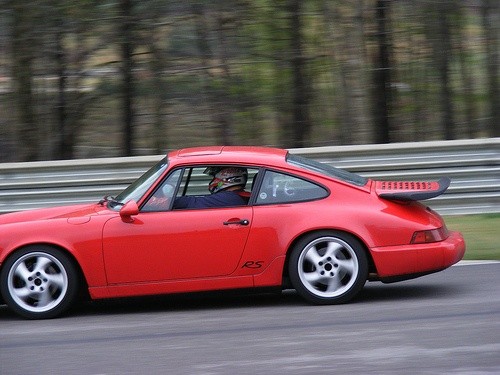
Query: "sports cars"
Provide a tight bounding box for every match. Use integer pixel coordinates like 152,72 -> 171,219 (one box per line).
0,141 -> 468,323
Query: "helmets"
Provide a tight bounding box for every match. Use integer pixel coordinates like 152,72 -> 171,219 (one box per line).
203,164 -> 246,195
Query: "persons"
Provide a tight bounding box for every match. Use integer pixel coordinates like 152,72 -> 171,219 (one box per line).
137,166 -> 253,210
